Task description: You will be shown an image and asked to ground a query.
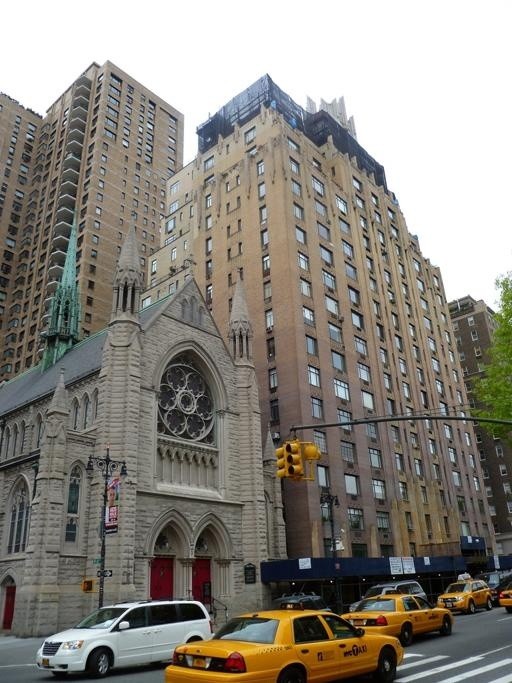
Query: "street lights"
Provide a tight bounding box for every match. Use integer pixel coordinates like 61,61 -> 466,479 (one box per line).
321,488 -> 343,616
86,443 -> 128,624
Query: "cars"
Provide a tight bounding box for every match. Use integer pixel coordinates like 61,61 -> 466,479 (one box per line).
275,571 -> 512,647
163,602 -> 404,683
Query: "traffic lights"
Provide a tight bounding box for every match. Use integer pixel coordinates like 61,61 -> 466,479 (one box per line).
275,440 -> 304,477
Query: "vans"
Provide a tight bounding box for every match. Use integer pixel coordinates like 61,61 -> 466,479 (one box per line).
34,599 -> 214,679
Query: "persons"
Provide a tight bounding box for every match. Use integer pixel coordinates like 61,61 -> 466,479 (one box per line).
105,486 -> 118,522
